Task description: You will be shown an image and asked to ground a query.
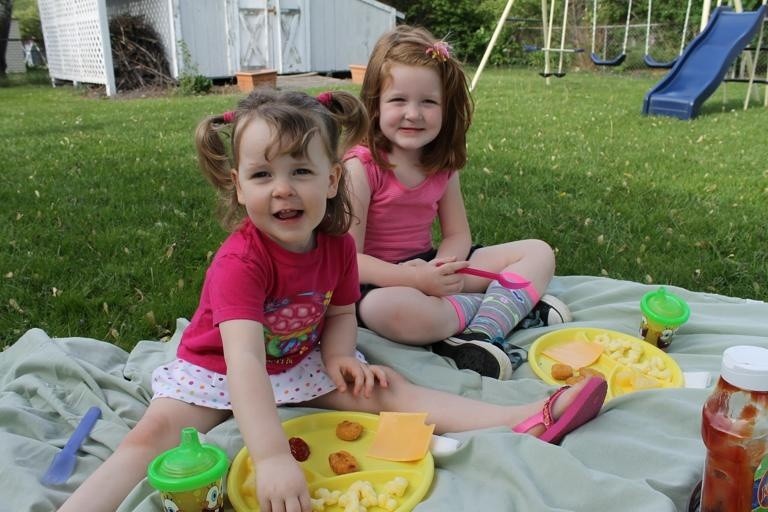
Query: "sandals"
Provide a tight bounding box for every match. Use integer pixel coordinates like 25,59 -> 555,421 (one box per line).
514,374 -> 608,444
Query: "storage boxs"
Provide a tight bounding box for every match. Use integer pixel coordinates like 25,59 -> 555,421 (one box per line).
348,63 -> 368,84
236,69 -> 277,94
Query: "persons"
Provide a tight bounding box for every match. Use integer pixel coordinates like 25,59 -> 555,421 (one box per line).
335,22 -> 573,380
57,88 -> 607,512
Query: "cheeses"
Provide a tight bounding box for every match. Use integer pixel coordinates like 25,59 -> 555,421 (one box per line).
368,411 -> 436,462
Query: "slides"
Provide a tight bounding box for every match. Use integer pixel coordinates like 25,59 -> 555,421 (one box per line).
643,3 -> 768,120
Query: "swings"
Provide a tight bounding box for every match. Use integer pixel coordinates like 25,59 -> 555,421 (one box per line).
644,0 -> 692,68
523,1 -> 586,77
590,0 -> 632,67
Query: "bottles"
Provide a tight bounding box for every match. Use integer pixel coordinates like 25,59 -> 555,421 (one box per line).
696,345 -> 767,512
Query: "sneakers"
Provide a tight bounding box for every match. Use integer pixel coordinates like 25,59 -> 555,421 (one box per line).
514,294 -> 573,330
430,330 -> 513,381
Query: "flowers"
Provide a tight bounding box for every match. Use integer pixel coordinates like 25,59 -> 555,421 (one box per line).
425,40 -> 450,63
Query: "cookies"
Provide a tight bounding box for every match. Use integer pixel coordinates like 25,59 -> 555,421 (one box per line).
596,334 -> 672,384
310,476 -> 409,512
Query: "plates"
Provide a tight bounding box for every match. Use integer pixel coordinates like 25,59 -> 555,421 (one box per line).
529,328 -> 685,404
226,409 -> 435,512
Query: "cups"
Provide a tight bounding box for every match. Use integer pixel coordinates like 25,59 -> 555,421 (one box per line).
148,424 -> 223,512
638,287 -> 689,350
